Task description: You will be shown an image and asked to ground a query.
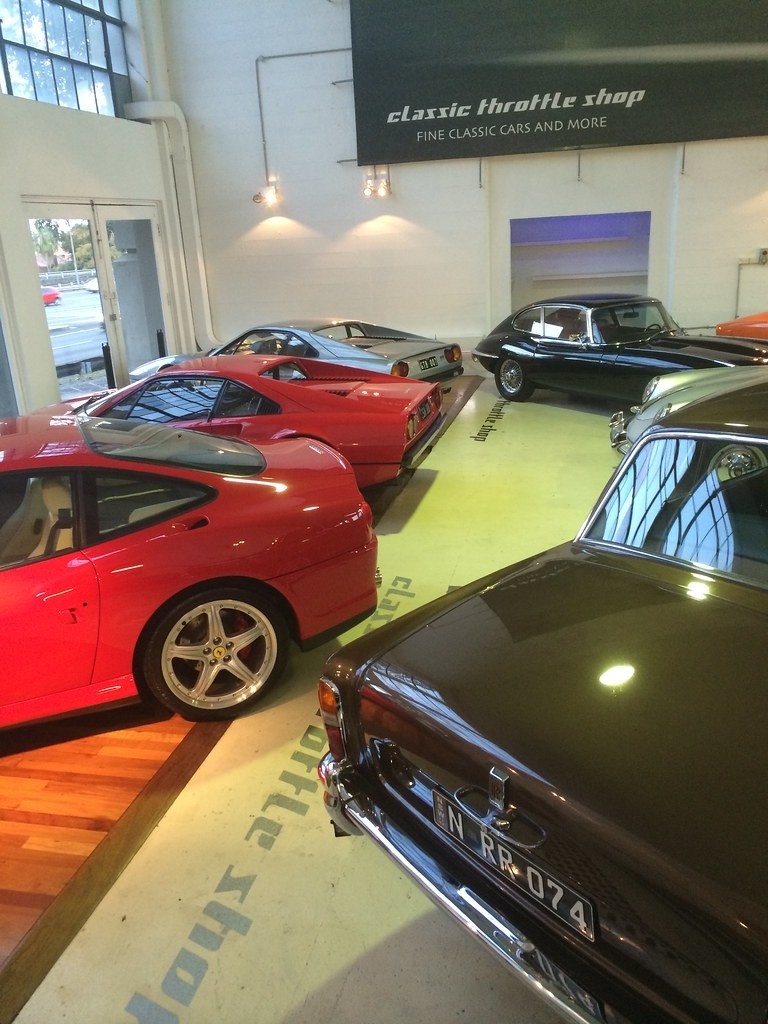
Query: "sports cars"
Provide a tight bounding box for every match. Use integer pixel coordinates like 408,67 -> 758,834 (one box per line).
0,317 -> 464,731
473,294 -> 768,404
319,312 -> 768,1024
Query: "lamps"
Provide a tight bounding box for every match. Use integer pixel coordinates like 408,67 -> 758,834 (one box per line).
378,179 -> 391,197
362,180 -> 377,197
252,192 -> 264,203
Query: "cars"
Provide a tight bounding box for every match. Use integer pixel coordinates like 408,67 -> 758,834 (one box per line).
83,276 -> 99,293
41,287 -> 62,307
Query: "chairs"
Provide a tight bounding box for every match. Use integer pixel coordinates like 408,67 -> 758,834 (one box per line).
248,334 -> 296,355
595,318 -> 612,339
0,476 -> 49,558
28,475 -> 74,559
